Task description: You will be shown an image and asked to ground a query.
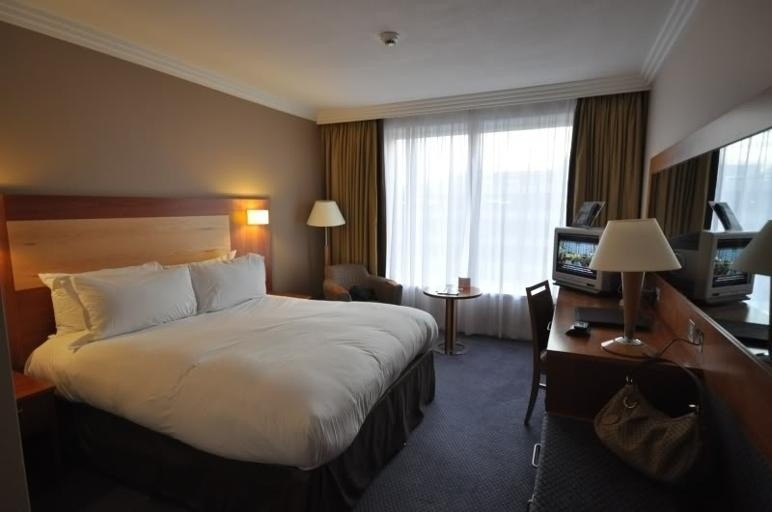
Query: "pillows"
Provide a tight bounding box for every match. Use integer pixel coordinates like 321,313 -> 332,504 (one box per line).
36,261 -> 160,341
189,252 -> 268,316
61,263 -> 197,349
169,248 -> 238,271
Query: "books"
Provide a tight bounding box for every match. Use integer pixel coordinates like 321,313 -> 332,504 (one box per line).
585,199 -> 607,229
458,277 -> 471,292
714,201 -> 743,231
437,285 -> 460,296
572,201 -> 599,227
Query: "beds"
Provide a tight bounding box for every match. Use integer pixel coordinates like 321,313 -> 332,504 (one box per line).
0,193 -> 440,512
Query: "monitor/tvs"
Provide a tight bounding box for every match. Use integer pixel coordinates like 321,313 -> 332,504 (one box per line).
673,229 -> 756,304
552,226 -> 617,295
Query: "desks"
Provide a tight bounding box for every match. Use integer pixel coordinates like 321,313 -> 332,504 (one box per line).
546,284 -> 699,417
421,282 -> 484,358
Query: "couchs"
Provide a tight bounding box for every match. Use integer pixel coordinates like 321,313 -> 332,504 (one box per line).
323,264 -> 404,306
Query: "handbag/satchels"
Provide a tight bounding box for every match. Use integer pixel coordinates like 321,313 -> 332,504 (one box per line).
594,379 -> 704,486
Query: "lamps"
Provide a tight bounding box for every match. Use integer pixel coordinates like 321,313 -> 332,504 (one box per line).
242,205 -> 273,229
729,217 -> 772,363
589,216 -> 682,358
307,198 -> 346,265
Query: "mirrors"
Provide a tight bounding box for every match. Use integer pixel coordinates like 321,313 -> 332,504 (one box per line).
646,80 -> 771,373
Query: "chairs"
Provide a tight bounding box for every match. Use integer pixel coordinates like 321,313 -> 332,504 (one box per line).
524,279 -> 555,429
